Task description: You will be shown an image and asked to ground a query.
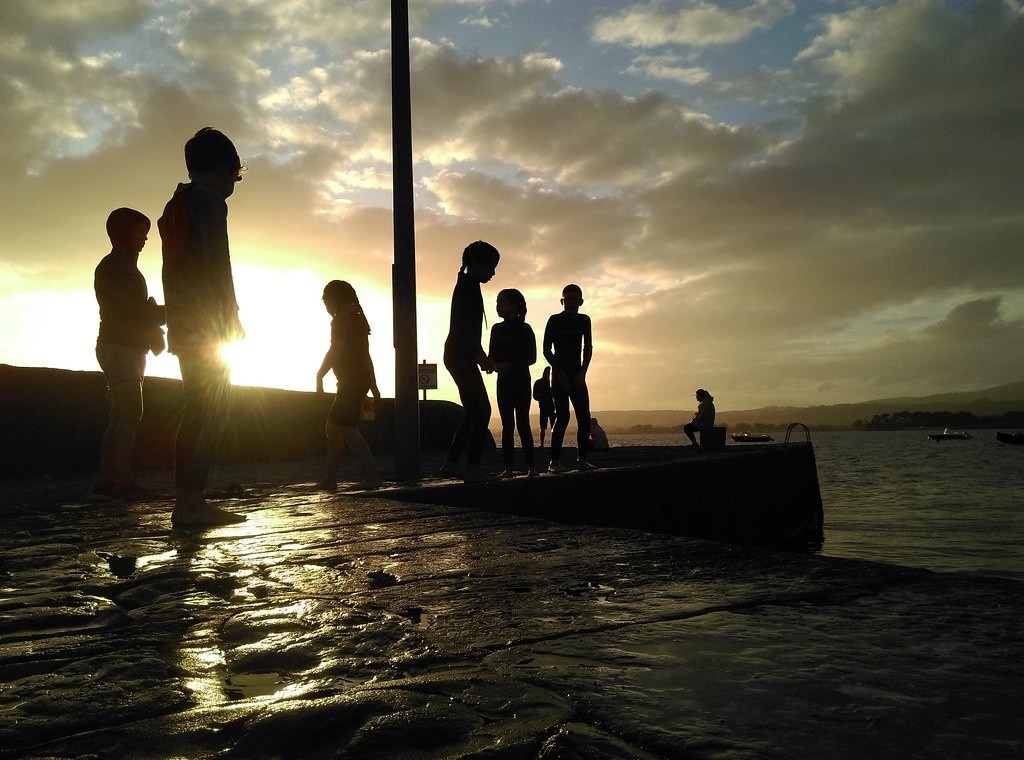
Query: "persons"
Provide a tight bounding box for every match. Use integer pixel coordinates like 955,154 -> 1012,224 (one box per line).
544,284 -> 597,472
683,389 -> 714,448
588,417 -> 609,450
158,128 -> 248,526
738,430 -> 750,437
94,207 -> 164,502
438,240 -> 499,479
311,280 -> 380,490
488,288 -> 538,479
534,366 -> 557,447
944,426 -> 950,435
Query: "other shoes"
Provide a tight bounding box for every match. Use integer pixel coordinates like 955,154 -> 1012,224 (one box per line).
497,467 -> 513,480
309,473 -> 337,492
686,443 -> 700,449
548,464 -> 561,473
529,466 -> 538,478
344,477 -> 376,491
94,481 -> 117,502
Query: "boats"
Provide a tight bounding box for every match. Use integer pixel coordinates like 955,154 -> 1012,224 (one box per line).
994,431 -> 1024,445
730,432 -> 774,443
927,433 -> 969,440
